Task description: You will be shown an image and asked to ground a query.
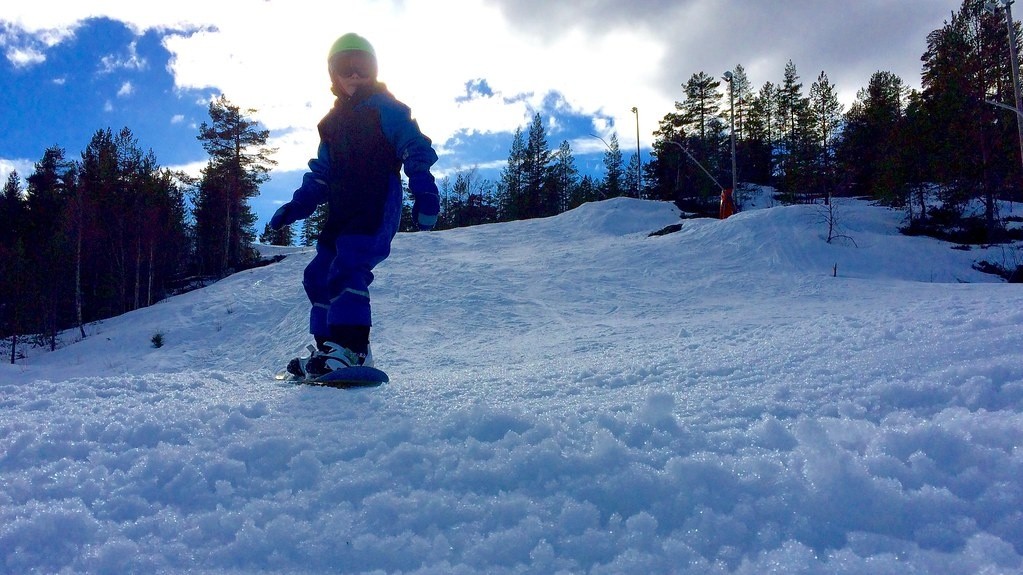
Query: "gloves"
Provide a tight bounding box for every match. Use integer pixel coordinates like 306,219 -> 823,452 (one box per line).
412,192 -> 440,231
270,200 -> 306,231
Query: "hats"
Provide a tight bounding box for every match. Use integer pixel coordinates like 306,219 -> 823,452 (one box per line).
328,32 -> 377,62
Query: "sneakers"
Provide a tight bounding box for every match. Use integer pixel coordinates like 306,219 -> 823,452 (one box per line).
305,341 -> 374,380
287,341 -> 324,380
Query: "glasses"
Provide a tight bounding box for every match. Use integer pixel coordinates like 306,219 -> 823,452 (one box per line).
335,59 -> 375,78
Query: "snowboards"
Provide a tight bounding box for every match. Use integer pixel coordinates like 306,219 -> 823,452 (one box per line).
284,364 -> 390,387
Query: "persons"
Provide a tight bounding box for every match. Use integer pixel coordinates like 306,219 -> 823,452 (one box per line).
719,188 -> 737,219
269,34 -> 441,380
